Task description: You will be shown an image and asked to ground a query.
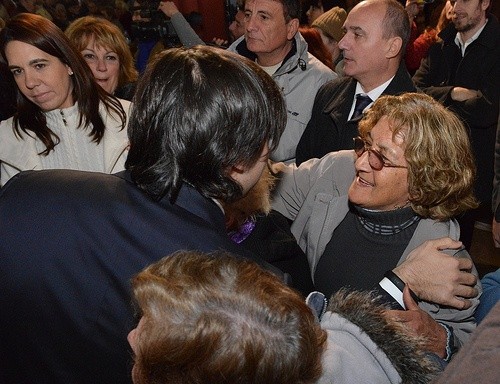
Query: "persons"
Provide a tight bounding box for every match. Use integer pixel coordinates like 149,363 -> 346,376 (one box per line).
0,0 -> 500,384
0,13 -> 133,187
255,93 -> 481,362
64,16 -> 139,96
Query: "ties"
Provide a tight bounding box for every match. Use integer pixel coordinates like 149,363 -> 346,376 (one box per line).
349,92 -> 371,121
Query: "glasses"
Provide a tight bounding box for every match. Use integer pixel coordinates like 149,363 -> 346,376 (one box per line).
354,135 -> 409,171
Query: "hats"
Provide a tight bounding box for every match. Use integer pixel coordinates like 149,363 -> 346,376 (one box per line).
312,6 -> 348,40
